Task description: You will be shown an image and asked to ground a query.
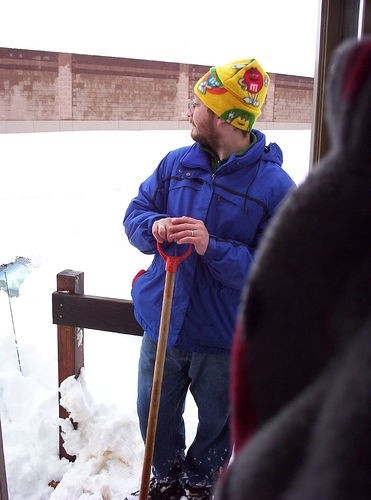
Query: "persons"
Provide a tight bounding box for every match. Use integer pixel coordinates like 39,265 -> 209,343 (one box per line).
121,57 -> 296,500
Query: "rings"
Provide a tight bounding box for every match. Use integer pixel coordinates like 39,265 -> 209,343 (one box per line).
159,225 -> 164,228
192,230 -> 194,237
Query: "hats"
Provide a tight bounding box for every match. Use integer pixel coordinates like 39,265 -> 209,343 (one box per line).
193,57 -> 272,132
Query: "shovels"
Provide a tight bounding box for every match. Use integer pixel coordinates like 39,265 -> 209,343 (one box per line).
140,241 -> 192,500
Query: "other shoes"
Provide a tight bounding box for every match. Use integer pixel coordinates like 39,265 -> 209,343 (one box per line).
124,476 -> 183,500
184,479 -> 213,500
